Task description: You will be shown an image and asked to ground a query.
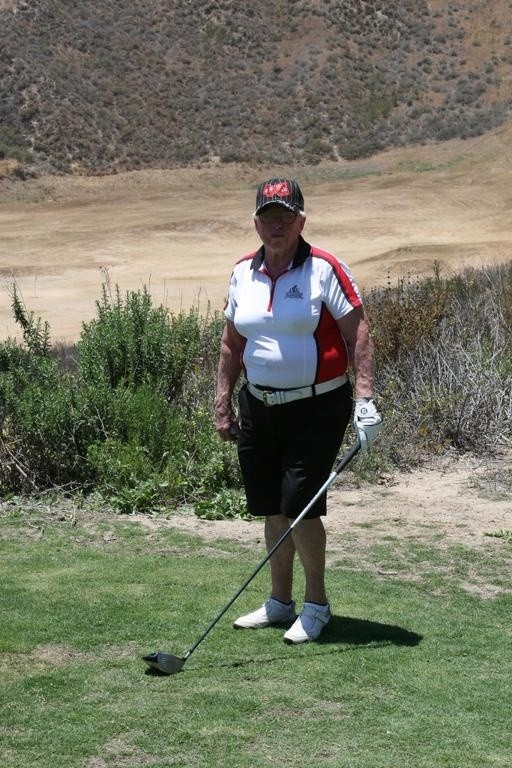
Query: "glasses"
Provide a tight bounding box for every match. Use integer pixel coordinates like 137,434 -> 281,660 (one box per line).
259,211 -> 300,225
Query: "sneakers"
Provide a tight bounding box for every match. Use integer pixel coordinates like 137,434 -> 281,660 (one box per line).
233,598 -> 296,629
283,602 -> 332,645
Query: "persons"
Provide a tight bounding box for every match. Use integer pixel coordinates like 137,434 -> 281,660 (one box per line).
213,179 -> 381,645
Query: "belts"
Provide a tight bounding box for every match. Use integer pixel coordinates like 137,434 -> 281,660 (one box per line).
246,375 -> 349,407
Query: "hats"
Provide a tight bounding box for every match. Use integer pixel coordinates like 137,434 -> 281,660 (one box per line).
255,179 -> 304,215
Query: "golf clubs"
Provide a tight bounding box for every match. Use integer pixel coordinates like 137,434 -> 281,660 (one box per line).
142,440 -> 361,675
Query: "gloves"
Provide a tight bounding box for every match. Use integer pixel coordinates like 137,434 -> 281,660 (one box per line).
353,397 -> 383,451
